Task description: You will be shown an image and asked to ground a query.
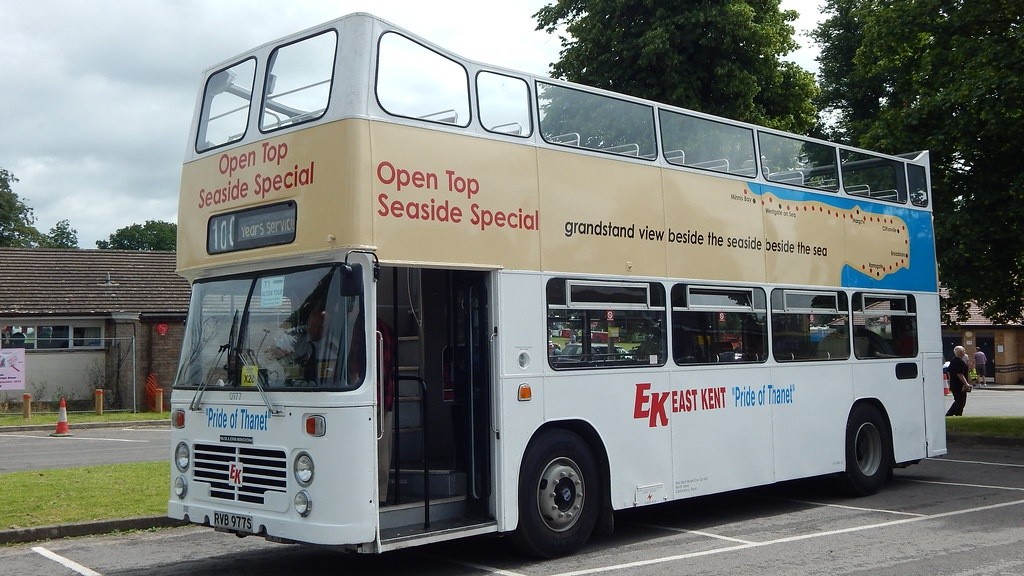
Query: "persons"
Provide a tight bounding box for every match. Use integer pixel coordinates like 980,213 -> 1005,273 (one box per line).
946,346 -> 971,417
335,297 -> 395,508
267,306 -> 332,388
973,347 -> 988,387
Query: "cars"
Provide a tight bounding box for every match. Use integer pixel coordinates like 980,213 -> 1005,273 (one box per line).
557,343 -> 633,368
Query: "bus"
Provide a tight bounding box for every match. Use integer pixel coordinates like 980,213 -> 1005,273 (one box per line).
167,11 -> 947,561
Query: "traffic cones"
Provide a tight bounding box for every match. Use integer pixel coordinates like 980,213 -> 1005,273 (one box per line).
49,397 -> 74,437
943,373 -> 950,395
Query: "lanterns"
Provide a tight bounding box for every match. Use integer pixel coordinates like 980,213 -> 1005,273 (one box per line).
156,324 -> 169,336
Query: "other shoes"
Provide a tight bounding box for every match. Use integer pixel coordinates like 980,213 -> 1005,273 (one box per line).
983,384 -> 987,387
976,383 -> 981,388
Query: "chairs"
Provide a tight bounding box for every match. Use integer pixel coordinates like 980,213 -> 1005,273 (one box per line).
699,350 -> 831,364
419,110 -> 902,206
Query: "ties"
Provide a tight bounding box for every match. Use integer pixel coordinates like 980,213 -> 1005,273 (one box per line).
303,341 -> 317,382
